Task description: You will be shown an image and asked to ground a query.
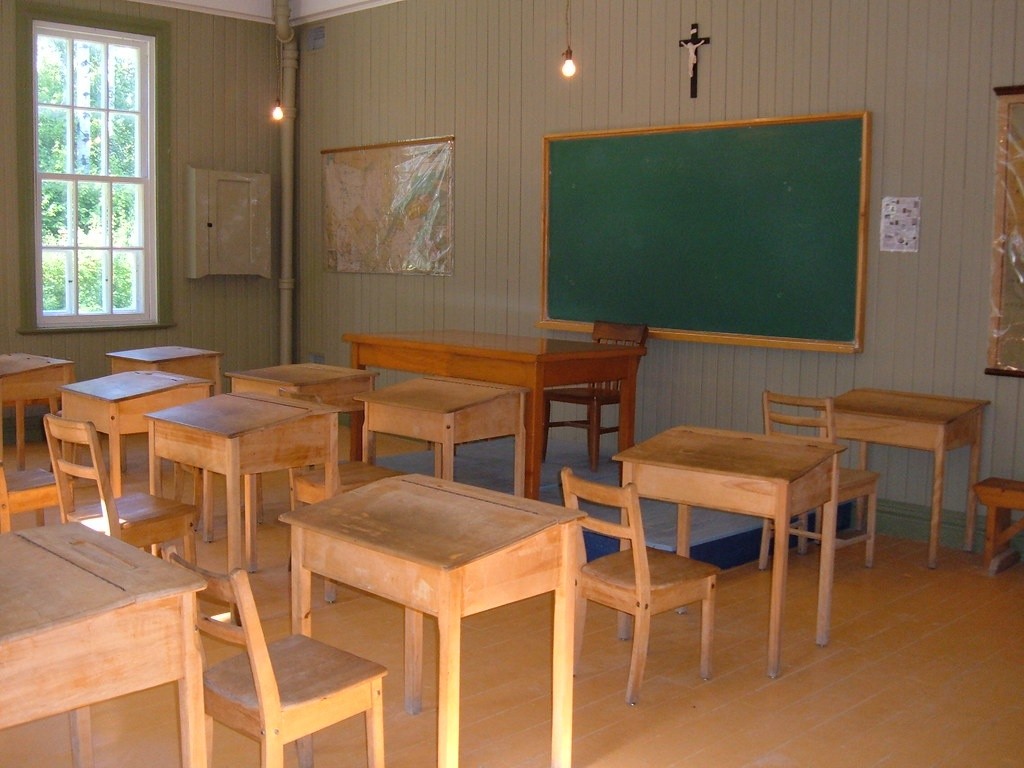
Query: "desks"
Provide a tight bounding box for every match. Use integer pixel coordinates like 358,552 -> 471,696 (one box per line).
813,387 -> 991,569
143,389 -> 341,625
0,352 -> 75,475
0,521 -> 207,768
224,362 -> 381,465
277,474 -> 589,768
611,425 -> 848,678
342,329 -> 648,500
353,377 -> 528,499
55,369 -> 216,499
105,345 -> 224,395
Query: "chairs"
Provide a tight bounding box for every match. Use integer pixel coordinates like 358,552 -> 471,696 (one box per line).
759,390 -> 879,570
161,370 -> 266,532
0,461 -> 75,534
159,544 -> 389,768
279,386 -> 412,571
972,476 -> 1024,572
42,413 -> 198,568
543,320 -> 651,472
561,465 -> 724,706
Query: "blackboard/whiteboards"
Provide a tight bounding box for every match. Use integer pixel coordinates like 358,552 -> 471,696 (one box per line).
540,110 -> 872,354
